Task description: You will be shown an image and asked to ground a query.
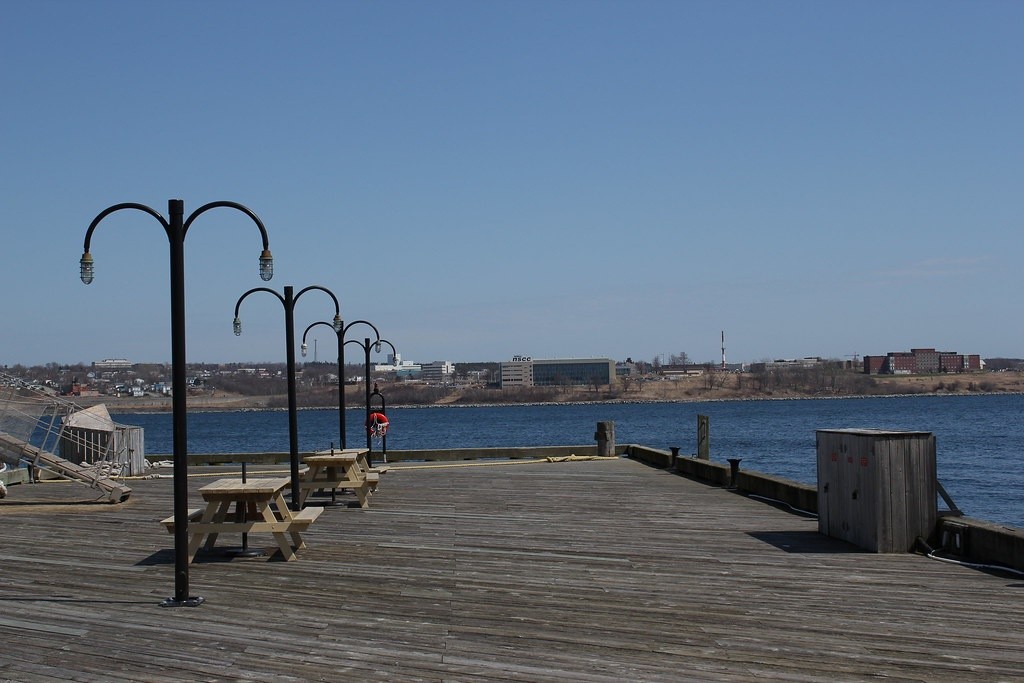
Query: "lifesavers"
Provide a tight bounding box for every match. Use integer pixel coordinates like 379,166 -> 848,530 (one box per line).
365,413 -> 390,437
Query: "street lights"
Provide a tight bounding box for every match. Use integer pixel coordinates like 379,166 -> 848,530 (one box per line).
338,339 -> 399,467
232,283 -> 345,512
299,319 -> 384,492
78,198 -> 276,607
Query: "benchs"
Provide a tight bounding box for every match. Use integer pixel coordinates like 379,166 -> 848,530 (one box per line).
160,506 -> 325,534
288,471 -> 378,489
360,466 -> 390,473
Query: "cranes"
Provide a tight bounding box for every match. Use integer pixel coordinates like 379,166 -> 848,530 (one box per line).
843,350 -> 860,370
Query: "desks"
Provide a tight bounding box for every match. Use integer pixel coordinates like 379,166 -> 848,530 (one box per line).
188,478 -> 306,565
300,453 -> 372,510
317,449 -> 379,493
